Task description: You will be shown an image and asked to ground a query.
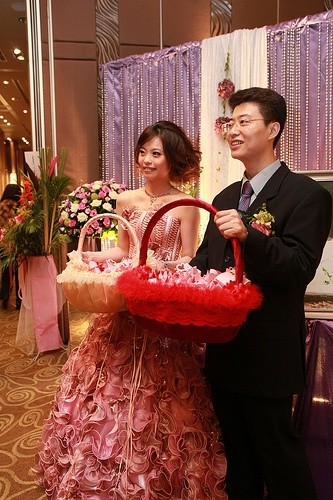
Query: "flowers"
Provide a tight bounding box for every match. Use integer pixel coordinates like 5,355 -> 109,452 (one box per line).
0,164 -> 72,267
252,204 -> 276,236
59,178 -> 128,239
218,77 -> 235,97
213,115 -> 233,136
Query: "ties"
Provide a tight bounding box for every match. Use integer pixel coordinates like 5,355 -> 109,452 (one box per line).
237,181 -> 253,219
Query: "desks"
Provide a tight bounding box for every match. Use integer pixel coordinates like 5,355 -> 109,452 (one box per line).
287,319 -> 333,500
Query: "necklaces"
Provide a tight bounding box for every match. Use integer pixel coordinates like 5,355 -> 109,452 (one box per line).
144,186 -> 175,206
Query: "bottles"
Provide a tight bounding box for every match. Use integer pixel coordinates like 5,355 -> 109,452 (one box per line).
100,230 -> 117,251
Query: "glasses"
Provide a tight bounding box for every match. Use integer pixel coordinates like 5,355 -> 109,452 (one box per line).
222,119 -> 265,132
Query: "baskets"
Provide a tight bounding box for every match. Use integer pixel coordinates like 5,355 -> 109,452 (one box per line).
121,198 -> 258,343
59,213 -> 139,313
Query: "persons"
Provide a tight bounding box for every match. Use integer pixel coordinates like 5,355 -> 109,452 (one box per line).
166,87 -> 333,500
38,122 -> 228,500
0,184 -> 22,308
